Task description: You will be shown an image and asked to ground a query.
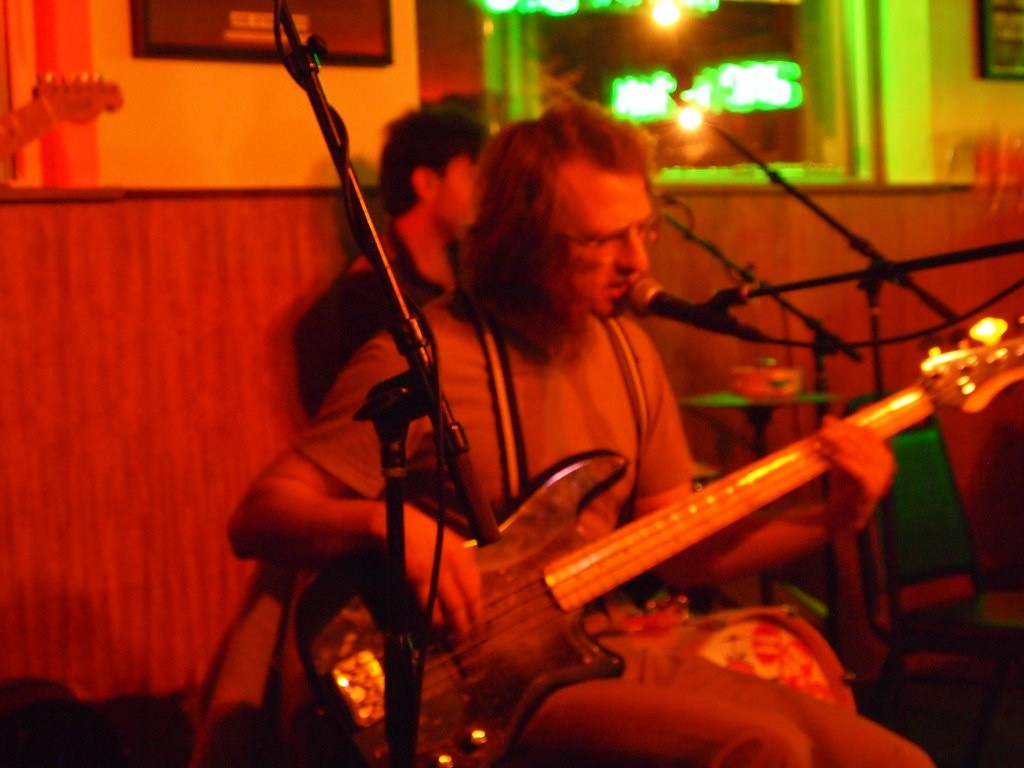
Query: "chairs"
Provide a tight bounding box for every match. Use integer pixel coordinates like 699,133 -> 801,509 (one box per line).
840,391 -> 1024,768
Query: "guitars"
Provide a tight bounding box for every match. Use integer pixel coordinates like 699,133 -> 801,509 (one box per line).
298,317 -> 1024,768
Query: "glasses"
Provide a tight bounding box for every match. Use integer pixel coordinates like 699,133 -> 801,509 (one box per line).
563,210 -> 663,257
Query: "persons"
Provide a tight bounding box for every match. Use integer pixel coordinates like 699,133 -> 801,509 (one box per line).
225,100 -> 938,768
296,101 -> 488,420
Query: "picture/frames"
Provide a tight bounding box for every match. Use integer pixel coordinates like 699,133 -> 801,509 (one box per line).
978,0 -> 1024,79
127,0 -> 394,68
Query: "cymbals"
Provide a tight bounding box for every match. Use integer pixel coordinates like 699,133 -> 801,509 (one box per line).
682,390 -> 831,412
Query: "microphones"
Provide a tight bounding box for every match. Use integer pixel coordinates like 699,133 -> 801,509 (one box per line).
625,276 -> 765,343
